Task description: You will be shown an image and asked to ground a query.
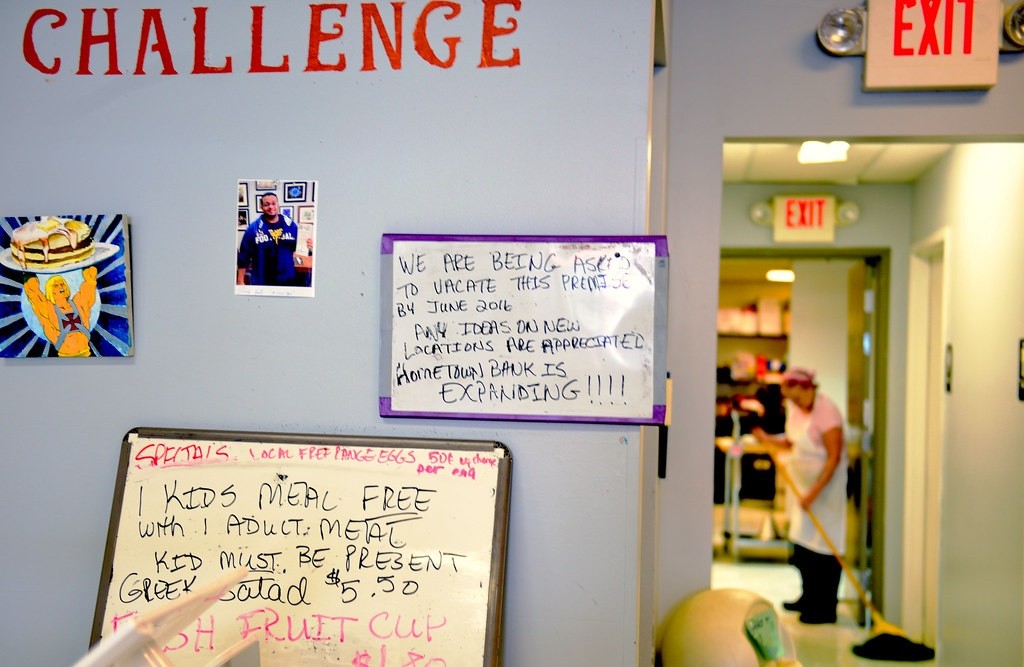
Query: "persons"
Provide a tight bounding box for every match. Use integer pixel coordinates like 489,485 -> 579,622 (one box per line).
238,193 -> 298,285
759,368 -> 848,624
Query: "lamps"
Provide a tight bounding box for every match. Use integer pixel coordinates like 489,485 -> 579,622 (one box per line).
999,0 -> 1024,53
836,200 -> 860,228
816,6 -> 869,57
748,200 -> 773,227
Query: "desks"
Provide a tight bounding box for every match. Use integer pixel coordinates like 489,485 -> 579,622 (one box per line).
714,435 -> 794,560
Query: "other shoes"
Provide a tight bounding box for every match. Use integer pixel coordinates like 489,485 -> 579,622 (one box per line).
800,612 -> 837,624
784,599 -> 806,612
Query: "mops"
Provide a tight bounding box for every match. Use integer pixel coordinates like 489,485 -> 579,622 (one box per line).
750,426 -> 937,662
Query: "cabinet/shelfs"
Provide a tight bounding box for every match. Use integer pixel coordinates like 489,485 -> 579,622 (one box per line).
713,333 -> 789,505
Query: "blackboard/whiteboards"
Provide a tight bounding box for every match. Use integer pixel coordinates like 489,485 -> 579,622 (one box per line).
87,425 -> 513,667
376,228 -> 669,426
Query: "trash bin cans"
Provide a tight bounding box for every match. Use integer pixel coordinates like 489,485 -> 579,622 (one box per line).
660,588 -> 797,667
